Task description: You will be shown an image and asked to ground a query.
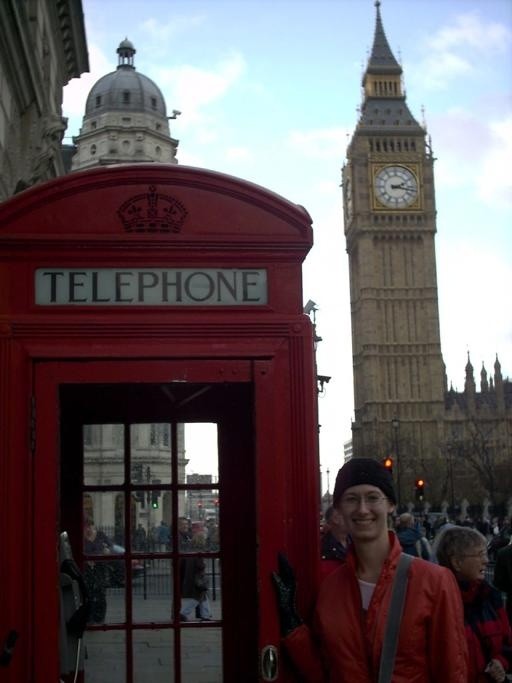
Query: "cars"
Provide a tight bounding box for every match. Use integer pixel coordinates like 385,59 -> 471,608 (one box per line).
104,543 -> 145,586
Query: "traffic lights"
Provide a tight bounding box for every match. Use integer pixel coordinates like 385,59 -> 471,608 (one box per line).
152,495 -> 158,508
131,463 -> 144,501
414,479 -> 425,505
384,456 -> 394,475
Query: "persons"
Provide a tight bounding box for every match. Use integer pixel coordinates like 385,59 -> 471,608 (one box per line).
321,507 -> 509,678
271,457 -> 471,682
87,506 -> 220,619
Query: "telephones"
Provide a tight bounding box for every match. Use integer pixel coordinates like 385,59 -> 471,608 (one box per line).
58,554 -> 93,643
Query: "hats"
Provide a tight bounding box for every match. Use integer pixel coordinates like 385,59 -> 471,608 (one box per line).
333,458 -> 396,508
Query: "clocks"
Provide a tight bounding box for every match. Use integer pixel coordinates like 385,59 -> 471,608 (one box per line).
372,163 -> 422,212
